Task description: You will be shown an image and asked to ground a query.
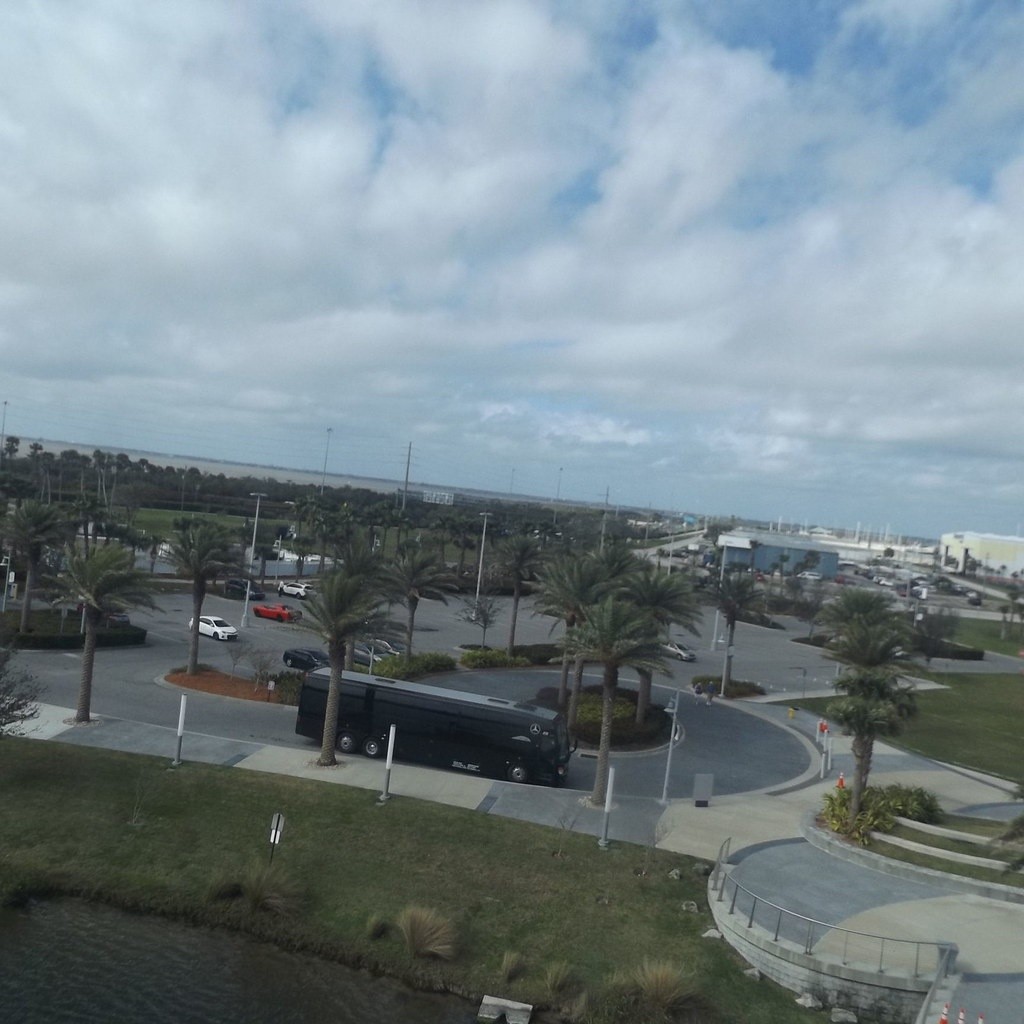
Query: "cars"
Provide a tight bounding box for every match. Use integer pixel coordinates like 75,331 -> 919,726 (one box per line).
746,562 -> 979,656
252,604 -> 302,621
660,640 -> 697,661
189,614 -> 239,640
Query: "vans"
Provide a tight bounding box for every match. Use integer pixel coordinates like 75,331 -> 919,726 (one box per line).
278,582 -> 317,600
225,578 -> 265,599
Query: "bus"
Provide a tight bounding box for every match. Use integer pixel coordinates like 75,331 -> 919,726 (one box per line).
295,666 -> 578,788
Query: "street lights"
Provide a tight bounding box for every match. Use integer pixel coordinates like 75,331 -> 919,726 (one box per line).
708,540 -> 735,644
241,492 -> 268,628
0,555 -> 10,613
273,535 -> 283,585
471,512 -> 493,620
662,685 -> 684,802
